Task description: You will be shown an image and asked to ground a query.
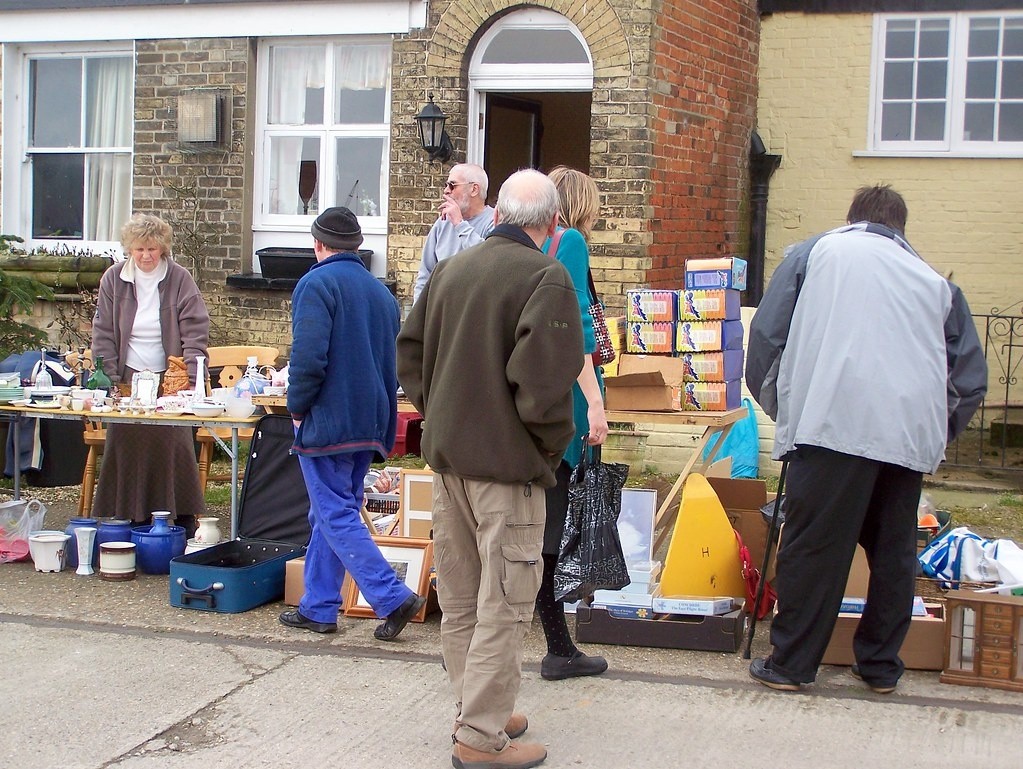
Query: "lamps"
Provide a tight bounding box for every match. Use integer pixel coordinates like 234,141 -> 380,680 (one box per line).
414,92 -> 453,164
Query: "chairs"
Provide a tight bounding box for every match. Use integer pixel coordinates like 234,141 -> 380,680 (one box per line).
75,350 -> 185,517
192,346 -> 279,526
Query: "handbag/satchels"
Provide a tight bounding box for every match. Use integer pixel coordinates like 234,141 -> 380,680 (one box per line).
703,398 -> 759,479
917,527 -> 1023,594
734,530 -> 777,620
553,433 -> 631,604
0,499 -> 48,564
550,227 -> 616,367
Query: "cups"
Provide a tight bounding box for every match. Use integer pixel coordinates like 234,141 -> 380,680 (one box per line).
58,388 -> 107,411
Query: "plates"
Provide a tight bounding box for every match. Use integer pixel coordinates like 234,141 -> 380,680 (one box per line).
157,410 -> 184,417
0,388 -> 32,407
27,404 -> 61,409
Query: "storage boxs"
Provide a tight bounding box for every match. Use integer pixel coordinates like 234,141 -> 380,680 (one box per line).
572,455 -> 950,673
601,257 -> 747,411
285,555 -> 351,611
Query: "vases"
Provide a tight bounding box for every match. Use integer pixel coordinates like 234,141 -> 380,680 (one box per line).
253,247 -> 374,281
28,512 -> 220,582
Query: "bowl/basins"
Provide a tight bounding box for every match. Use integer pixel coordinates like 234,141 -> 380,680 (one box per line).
191,406 -> 224,417
225,405 -> 256,418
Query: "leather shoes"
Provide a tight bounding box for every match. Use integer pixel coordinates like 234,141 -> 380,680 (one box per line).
374,592 -> 427,640
279,610 -> 337,632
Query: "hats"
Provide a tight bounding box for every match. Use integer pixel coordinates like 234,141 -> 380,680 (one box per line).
311,206 -> 364,249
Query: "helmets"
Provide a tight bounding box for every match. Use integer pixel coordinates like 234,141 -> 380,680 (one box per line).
920,514 -> 938,527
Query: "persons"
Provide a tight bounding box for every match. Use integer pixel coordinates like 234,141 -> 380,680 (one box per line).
439,163 -> 611,681
89,211 -> 210,541
161,355 -> 190,395
275,206 -> 426,641
745,183 -> 988,693
394,169 -> 585,769
411,162 -> 496,307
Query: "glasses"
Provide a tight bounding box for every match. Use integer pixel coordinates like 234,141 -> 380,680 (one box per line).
444,182 -> 475,191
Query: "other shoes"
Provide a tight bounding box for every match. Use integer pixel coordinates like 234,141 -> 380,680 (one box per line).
541,649 -> 609,679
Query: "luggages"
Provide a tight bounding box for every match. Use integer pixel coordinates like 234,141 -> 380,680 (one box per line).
169,414 -> 315,614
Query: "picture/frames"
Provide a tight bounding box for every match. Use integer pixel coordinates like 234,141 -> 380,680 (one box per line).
343,468 -> 435,624
618,488 -> 658,560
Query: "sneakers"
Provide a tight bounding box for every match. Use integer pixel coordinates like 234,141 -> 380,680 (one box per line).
452,714 -> 528,738
748,655 -> 799,689
451,742 -> 548,769
851,662 -> 896,691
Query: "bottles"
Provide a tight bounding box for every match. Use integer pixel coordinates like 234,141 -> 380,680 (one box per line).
35,365 -> 52,391
86,355 -> 114,397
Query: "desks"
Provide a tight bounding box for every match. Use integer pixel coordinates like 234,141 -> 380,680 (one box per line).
1,405 -> 262,543
